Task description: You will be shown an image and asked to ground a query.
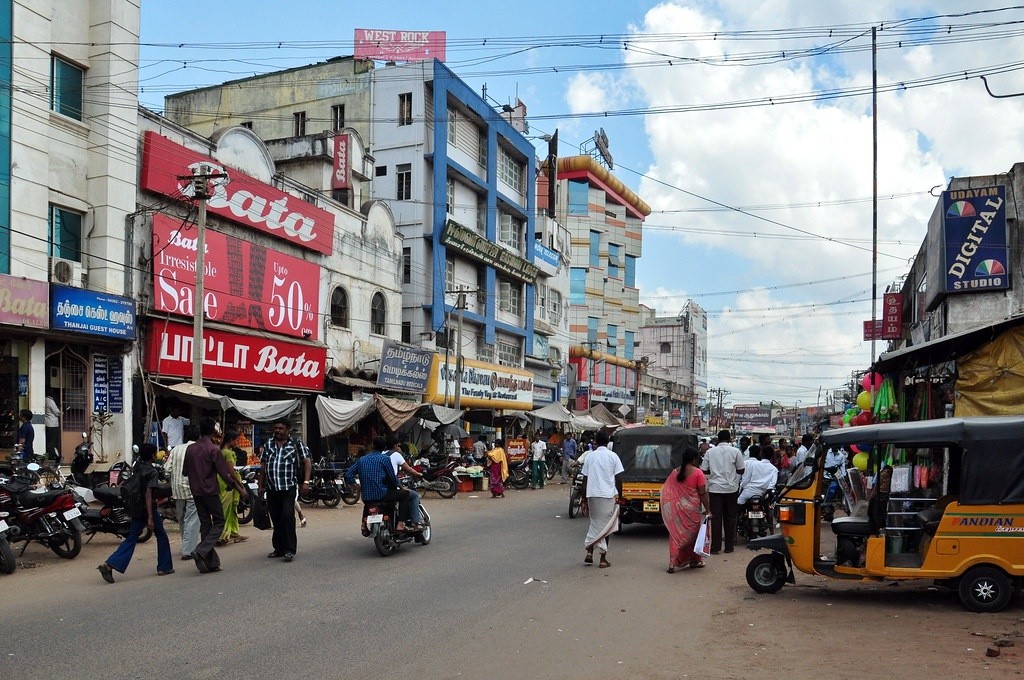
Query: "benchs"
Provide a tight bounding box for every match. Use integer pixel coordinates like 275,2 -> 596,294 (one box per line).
917,449 -> 967,533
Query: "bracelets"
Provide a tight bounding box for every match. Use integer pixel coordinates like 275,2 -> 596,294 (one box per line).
303,481 -> 310,485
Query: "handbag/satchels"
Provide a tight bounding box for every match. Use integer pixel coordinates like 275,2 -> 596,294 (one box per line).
693,514 -> 711,557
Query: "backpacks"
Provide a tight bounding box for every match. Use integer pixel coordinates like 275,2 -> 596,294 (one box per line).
120,468 -> 158,516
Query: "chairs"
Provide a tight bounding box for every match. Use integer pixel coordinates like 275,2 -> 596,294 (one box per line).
829,464 -> 895,533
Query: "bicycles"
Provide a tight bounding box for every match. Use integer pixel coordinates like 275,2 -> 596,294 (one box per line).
0,444 -> 66,485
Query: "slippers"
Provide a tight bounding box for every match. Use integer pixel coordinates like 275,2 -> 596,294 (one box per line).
396,527 -> 415,532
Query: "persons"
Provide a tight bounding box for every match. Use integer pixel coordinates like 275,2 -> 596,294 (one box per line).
163,424 -> 199,561
257,417 -> 312,559
162,405 -> 818,545
71,441 -> 94,487
16,409 -> 35,462
182,417 -> 248,573
343,436 -> 416,538
821,444 -> 849,511
382,436 -> 427,533
701,429 -> 745,555
98,444 -> 176,583
46,387 -> 66,459
660,446 -> 712,574
580,431 -> 625,568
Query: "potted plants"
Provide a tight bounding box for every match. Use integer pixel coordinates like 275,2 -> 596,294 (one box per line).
89,411 -> 114,464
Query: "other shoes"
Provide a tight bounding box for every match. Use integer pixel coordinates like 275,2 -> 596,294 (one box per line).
585,557 -> 593,565
299,515 -> 307,527
181,555 -> 193,561
158,568 -> 177,575
97,563 -> 114,584
191,551 -> 222,572
690,561 -> 704,568
666,567 -> 675,573
600,561 -> 611,568
413,523 -> 427,532
268,552 -> 294,562
234,535 -> 248,543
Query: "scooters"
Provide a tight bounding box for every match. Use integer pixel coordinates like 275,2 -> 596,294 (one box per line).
67,432 -> 129,490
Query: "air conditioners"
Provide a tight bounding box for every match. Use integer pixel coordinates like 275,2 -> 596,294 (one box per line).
47,253 -> 88,286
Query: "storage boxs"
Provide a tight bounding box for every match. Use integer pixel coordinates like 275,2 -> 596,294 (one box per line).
473,477 -> 490,492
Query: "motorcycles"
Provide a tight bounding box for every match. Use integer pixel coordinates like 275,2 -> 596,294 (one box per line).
399,453 -> 462,499
0,463 -> 153,574
569,454 -> 587,518
299,456 -> 360,508
508,460 -> 529,489
117,445 -> 178,523
364,474 -> 432,556
746,415 -> 1024,612
611,426 -> 698,535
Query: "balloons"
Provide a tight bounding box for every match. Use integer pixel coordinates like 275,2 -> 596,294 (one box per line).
837,404 -> 871,428
850,443 -> 871,470
856,391 -> 872,409
862,372 -> 883,392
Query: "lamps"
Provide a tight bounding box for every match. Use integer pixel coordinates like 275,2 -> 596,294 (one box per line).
493,102 -> 515,113
525,134 -> 551,142
